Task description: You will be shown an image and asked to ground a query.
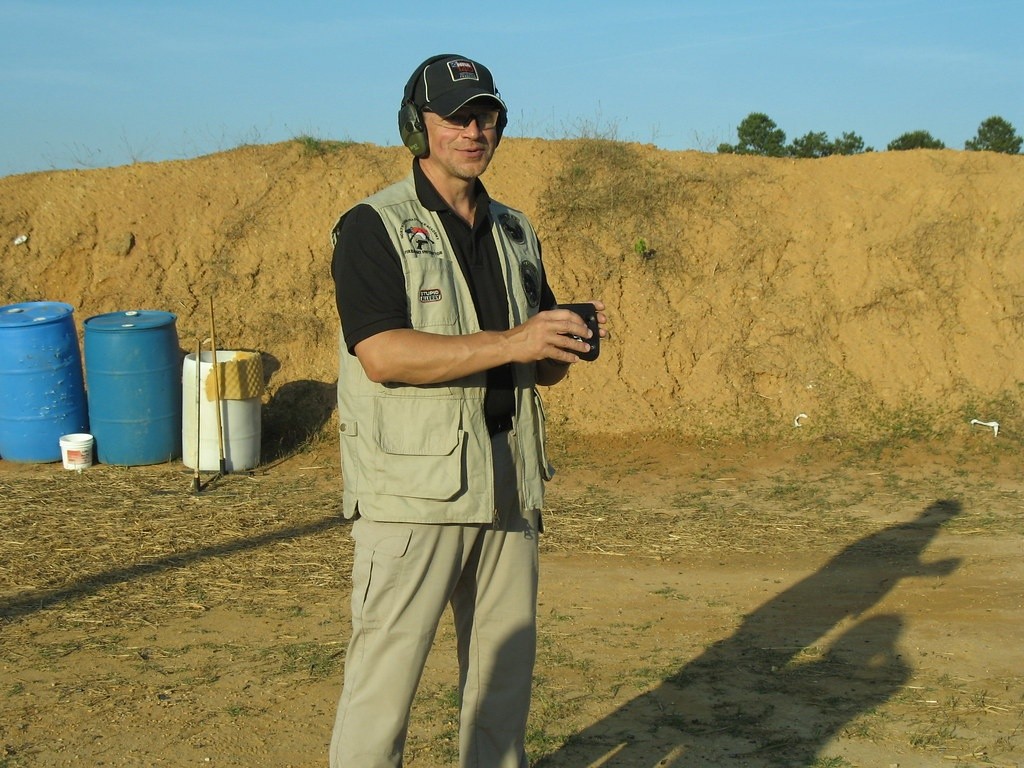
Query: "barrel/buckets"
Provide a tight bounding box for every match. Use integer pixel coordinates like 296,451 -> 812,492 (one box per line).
0,301 -> 88,464
79,309 -> 181,466
58,432 -> 95,470
182,351 -> 265,469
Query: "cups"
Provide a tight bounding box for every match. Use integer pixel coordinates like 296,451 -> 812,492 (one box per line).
548,303 -> 600,366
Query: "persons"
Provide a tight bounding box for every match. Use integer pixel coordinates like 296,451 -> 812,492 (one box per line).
331,54 -> 608,768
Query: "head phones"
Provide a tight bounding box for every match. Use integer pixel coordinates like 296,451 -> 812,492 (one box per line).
396,55 -> 465,158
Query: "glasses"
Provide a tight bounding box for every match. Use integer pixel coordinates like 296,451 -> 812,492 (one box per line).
422,106 -> 501,130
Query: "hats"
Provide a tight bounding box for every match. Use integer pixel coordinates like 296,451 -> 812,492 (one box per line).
404,53 -> 508,118
